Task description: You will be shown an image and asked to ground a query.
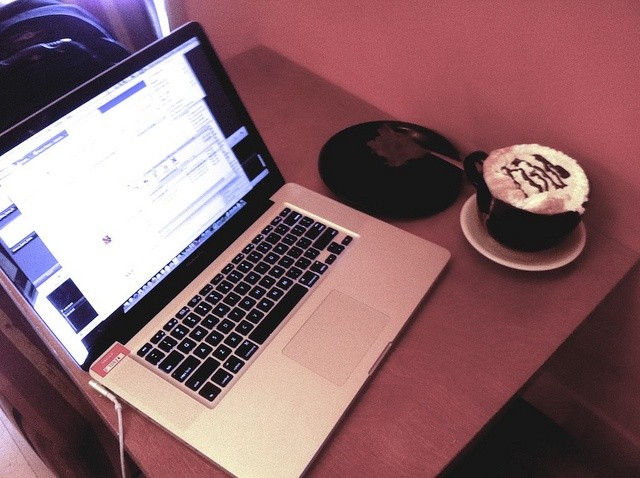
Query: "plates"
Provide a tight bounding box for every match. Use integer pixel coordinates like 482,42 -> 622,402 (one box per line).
460,194 -> 587,272
317,119 -> 460,219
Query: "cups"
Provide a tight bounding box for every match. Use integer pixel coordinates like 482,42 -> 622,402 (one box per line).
464,148 -> 590,251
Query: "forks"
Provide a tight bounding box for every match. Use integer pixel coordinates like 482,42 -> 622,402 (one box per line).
376,125 -> 467,174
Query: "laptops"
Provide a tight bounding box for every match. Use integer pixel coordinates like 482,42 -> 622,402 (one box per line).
0,20 -> 452,478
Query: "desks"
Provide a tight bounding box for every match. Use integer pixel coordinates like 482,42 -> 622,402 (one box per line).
0,44 -> 640,478
